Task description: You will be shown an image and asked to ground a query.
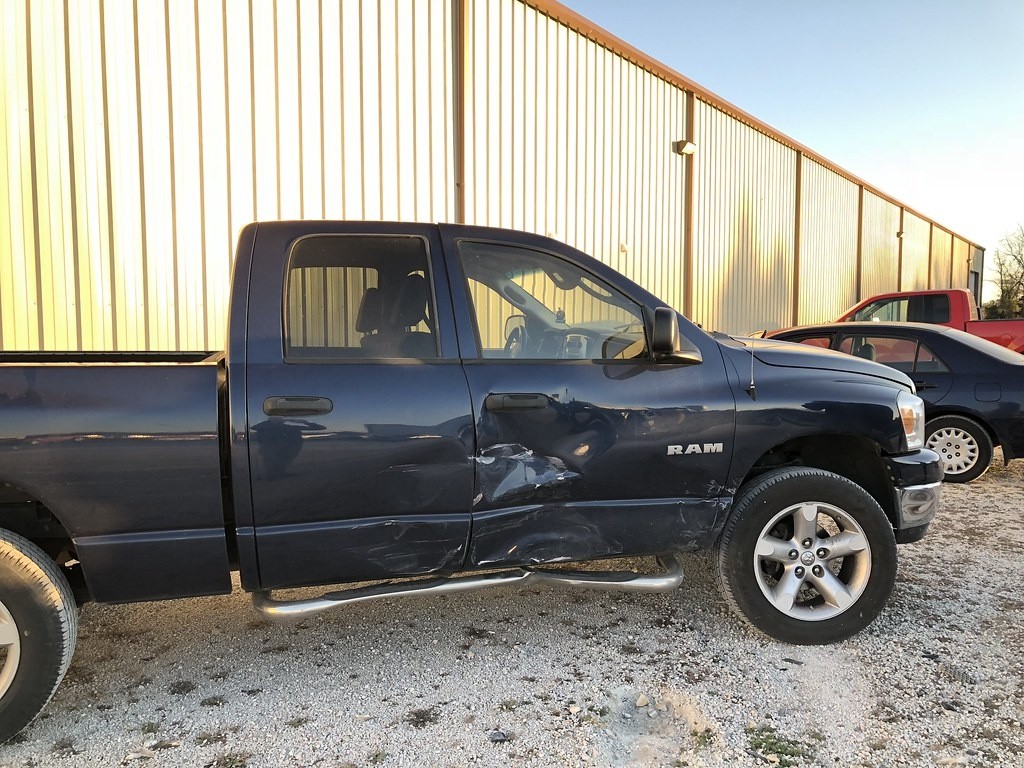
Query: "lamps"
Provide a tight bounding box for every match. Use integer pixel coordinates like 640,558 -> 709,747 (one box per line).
677,140 -> 697,156
967,259 -> 973,263
897,232 -> 906,237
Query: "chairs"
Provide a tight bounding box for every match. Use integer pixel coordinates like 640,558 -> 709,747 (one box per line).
355,287 -> 384,347
394,275 -> 436,359
856,344 -> 876,362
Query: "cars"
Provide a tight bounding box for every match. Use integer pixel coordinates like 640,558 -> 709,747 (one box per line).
743,321 -> 1024,486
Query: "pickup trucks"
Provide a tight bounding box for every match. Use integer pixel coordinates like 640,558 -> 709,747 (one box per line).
1,221 -> 945,748
749,290 -> 1024,364
